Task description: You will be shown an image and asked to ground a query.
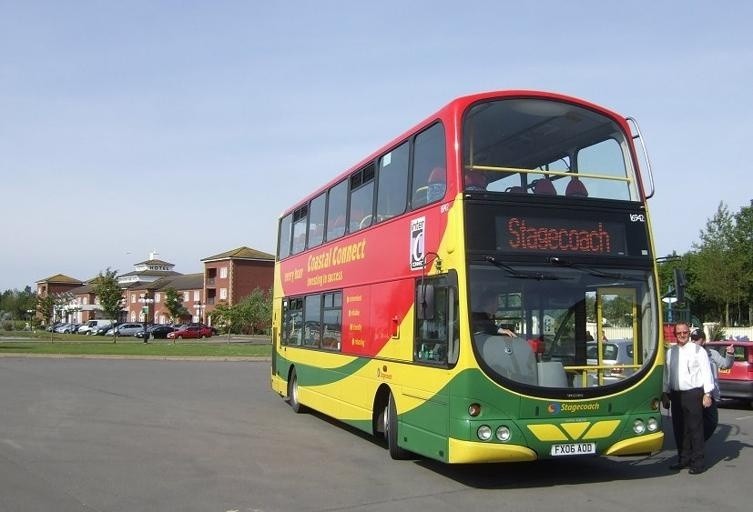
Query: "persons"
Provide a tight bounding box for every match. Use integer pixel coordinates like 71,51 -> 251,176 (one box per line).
469,290 -> 518,338
661,320 -> 715,475
688,328 -> 735,445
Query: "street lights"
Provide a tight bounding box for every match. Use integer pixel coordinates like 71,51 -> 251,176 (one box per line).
137,293 -> 154,344
26,309 -> 32,331
193,301 -> 205,341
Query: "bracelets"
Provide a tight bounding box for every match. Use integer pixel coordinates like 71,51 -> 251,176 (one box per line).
704,393 -> 711,398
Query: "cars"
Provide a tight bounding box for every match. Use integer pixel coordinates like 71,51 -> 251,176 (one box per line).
586,338 -> 634,387
702,340 -> 752,411
45,320 -> 212,340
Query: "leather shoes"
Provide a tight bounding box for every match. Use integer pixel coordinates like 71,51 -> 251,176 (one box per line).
689,468 -> 706,474
671,463 -> 684,469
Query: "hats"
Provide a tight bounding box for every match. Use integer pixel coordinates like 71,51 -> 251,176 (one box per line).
690,329 -> 705,339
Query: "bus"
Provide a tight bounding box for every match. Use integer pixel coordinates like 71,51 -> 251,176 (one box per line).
269,89 -> 685,479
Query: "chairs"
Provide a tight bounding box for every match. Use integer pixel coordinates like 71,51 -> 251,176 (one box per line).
427,167 -> 488,204
292,208 -> 383,255
282,300 -> 340,348
510,177 -> 588,196
